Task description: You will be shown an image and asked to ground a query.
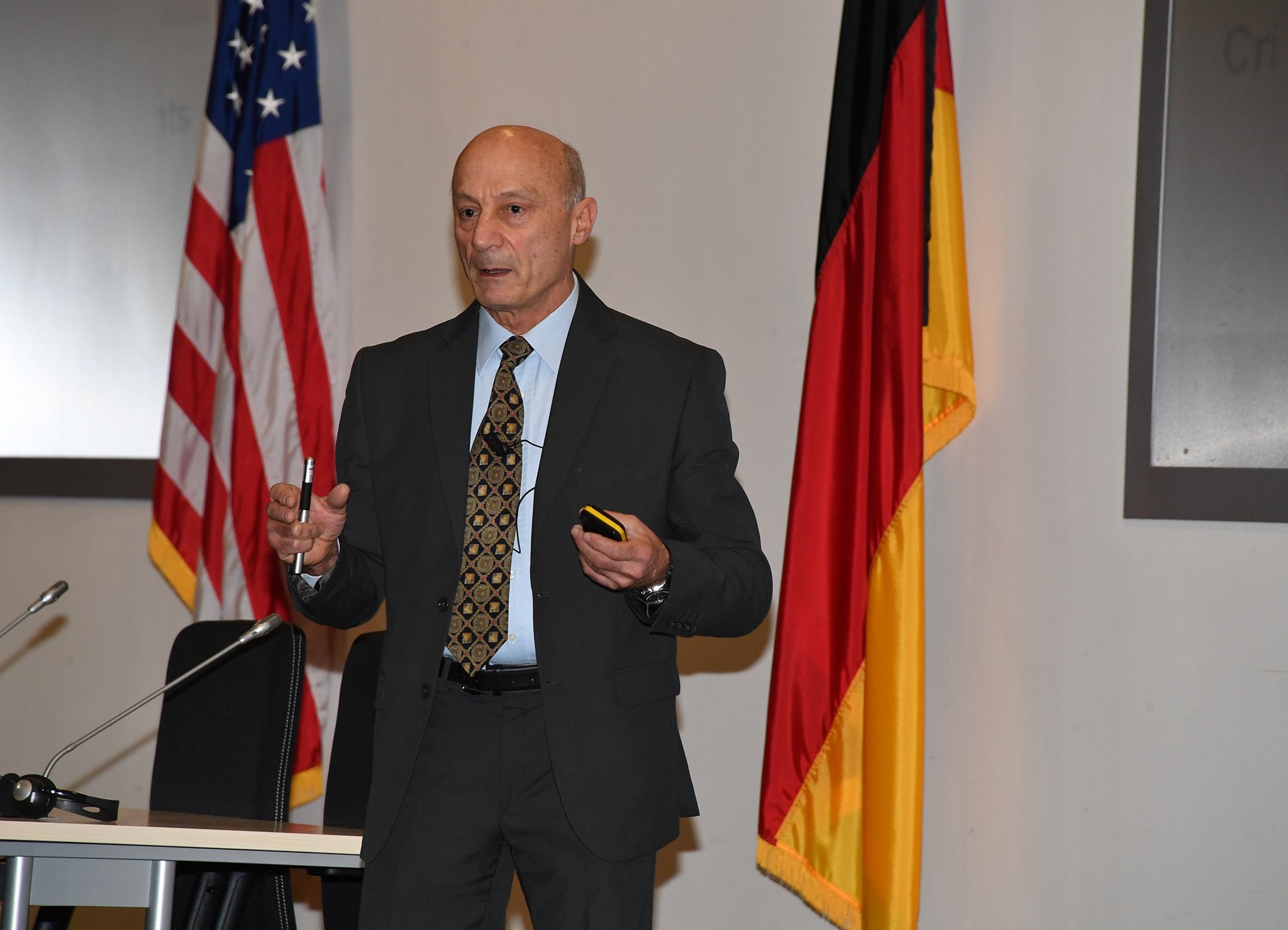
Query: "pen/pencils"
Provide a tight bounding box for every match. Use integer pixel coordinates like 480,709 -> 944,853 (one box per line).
290,456 -> 316,578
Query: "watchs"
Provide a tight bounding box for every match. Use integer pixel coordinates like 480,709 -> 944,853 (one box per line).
630,554 -> 674,603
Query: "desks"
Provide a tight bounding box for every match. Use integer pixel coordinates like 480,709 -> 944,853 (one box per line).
1,803 -> 364,929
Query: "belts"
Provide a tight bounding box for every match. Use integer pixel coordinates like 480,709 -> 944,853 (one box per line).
438,656 -> 541,695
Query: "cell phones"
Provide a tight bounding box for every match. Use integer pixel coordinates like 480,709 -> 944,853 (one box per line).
581,503 -> 627,543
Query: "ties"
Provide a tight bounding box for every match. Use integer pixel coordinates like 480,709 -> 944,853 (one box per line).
447,335 -> 534,676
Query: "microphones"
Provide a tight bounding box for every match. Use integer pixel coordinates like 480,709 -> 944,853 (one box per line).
0,580 -> 68,638
43,613 -> 283,778
492,441 -> 509,457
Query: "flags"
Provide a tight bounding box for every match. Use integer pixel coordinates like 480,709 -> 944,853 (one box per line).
758,0 -> 980,929
149,0 -> 342,811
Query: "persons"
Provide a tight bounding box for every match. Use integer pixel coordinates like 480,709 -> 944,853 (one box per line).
265,124 -> 773,930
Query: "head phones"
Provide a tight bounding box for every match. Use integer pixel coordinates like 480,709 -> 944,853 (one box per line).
0,771 -> 119,823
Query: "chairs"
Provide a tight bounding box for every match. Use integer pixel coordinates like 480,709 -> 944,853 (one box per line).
318,630 -> 385,929
144,621 -> 306,929
